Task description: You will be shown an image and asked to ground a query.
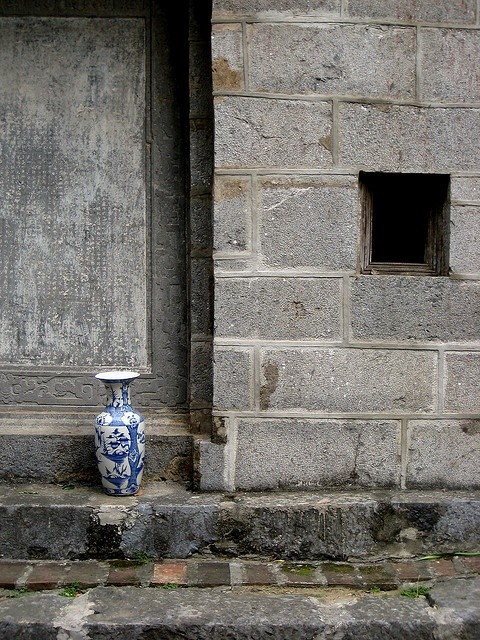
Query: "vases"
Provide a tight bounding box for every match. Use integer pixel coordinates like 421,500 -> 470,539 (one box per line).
94,371 -> 146,497
